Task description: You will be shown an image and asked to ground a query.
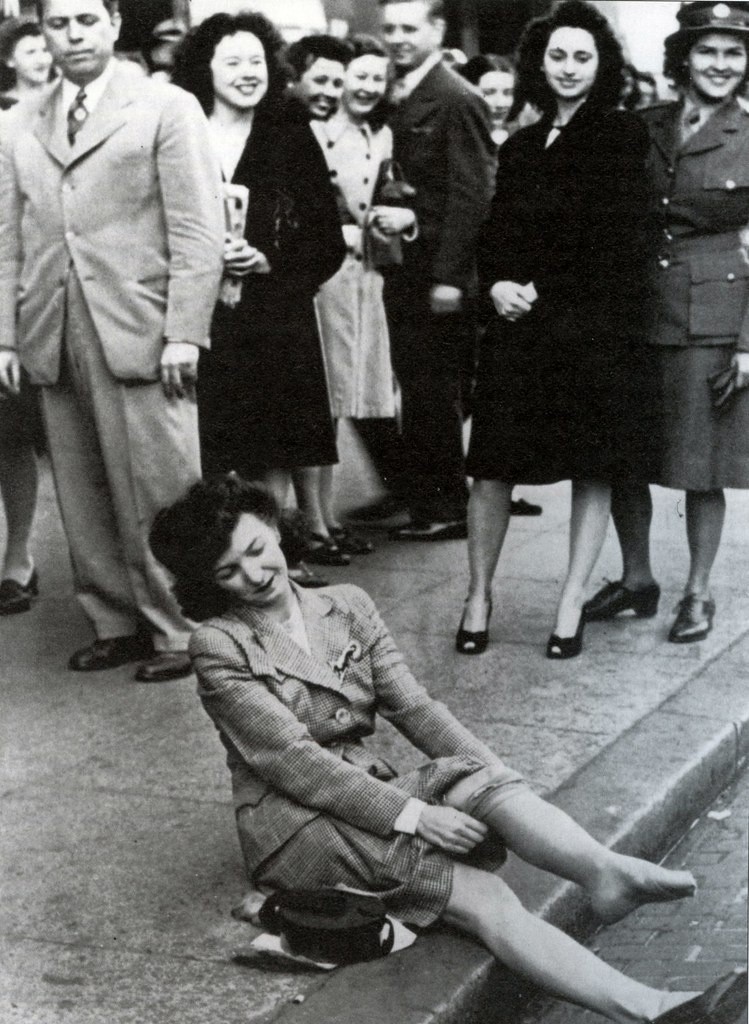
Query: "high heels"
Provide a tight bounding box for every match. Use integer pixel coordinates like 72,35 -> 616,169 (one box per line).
545,618 -> 585,659
327,526 -> 374,555
0,567 -> 39,616
455,597 -> 492,655
298,526 -> 352,566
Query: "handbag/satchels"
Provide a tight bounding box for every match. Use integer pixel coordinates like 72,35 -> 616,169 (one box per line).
258,887 -> 394,966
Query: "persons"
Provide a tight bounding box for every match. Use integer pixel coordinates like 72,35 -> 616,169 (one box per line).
145,475 -> 749,1023
1,0 -> 749,675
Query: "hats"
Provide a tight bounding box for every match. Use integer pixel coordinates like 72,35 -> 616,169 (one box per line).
664,1 -> 749,50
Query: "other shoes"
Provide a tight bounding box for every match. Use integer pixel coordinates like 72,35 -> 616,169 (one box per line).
508,498 -> 542,515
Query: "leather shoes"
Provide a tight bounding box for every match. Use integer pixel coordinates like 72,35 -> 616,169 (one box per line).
287,560 -> 328,586
384,518 -> 468,541
643,966 -> 748,1024
669,594 -> 716,643
68,635 -> 147,670
342,495 -> 412,530
135,653 -> 196,681
582,581 -> 660,620
